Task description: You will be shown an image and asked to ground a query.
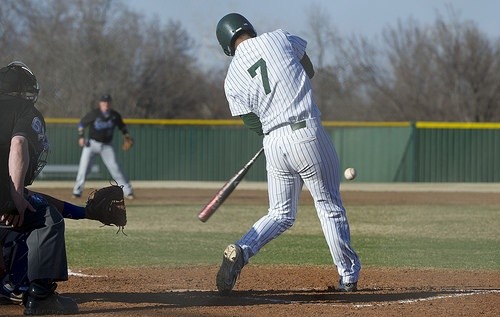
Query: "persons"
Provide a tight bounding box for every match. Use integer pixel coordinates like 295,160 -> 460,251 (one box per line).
0,61 -> 127,315
216,14 -> 361,295
72,94 -> 135,200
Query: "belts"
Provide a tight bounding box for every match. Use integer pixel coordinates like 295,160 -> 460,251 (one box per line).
263,120 -> 306,137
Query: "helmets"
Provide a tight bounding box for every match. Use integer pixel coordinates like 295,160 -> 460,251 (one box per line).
217,13 -> 257,56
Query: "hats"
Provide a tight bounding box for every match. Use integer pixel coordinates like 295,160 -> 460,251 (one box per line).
101,95 -> 111,101
0,66 -> 40,95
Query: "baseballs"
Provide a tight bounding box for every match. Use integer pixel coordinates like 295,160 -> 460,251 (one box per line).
343,167 -> 356,180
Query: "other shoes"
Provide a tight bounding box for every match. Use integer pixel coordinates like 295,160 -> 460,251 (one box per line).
72,194 -> 80,198
127,194 -> 135,200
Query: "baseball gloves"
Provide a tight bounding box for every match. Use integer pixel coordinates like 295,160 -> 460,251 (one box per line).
86,185 -> 128,227
122,138 -> 134,151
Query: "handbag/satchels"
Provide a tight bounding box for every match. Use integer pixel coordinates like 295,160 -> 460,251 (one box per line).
0,176 -> 36,231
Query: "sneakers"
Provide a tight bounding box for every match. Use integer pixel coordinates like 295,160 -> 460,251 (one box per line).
24,282 -> 78,315
0,275 -> 29,306
216,243 -> 245,297
334,278 -> 357,293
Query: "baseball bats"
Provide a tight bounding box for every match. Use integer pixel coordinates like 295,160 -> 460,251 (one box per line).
197,145 -> 265,224
